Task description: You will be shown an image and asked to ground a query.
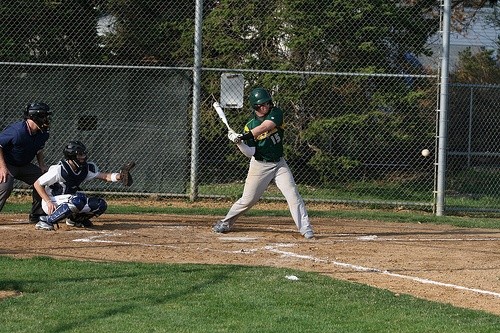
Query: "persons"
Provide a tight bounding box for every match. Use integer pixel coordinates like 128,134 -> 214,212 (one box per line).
34,140 -> 130,229
212,88 -> 314,238
0,101 -> 52,222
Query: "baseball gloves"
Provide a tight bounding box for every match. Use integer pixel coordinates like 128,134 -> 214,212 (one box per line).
121,161 -> 135,186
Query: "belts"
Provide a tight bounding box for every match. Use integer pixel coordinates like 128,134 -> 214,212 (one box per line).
253,155 -> 281,163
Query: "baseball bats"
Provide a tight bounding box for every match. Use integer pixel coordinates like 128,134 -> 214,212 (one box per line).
213,101 -> 239,143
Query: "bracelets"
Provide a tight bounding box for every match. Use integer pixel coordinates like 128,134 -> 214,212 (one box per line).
111,173 -> 120,182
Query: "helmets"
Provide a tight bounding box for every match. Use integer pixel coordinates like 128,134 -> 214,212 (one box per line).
64,141 -> 86,160
25,103 -> 52,134
250,88 -> 271,108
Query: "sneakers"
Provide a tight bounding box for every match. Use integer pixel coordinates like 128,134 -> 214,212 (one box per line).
304,230 -> 314,239
214,223 -> 224,233
66,218 -> 93,228
35,219 -> 54,231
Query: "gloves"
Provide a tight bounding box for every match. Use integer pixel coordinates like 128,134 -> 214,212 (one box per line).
228,132 -> 243,143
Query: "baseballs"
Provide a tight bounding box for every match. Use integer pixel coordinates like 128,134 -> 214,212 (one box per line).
422,149 -> 430,156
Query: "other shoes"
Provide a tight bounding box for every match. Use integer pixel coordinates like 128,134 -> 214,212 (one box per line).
28,214 -> 45,223
